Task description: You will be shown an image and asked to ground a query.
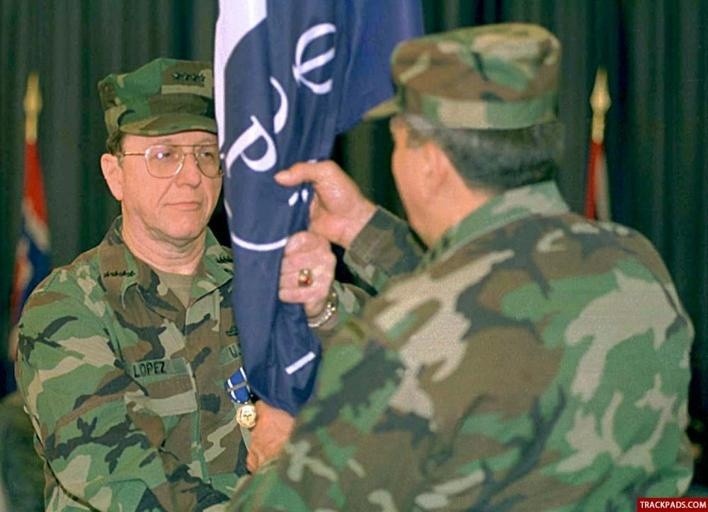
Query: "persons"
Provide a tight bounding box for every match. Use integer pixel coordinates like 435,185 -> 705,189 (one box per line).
200,19 -> 700,512
9,57 -> 372,512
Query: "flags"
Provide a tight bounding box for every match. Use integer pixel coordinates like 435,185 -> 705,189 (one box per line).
581,65 -> 614,225
209,0 -> 426,420
3,76 -> 55,396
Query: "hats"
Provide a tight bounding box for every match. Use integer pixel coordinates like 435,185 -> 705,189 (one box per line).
97,57 -> 218,136
360,22 -> 564,128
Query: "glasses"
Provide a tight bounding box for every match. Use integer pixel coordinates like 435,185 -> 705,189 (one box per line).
117,142 -> 221,179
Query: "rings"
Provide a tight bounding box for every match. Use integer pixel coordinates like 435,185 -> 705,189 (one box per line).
298,268 -> 311,288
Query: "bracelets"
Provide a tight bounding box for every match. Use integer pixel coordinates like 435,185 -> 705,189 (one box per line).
304,298 -> 337,330
